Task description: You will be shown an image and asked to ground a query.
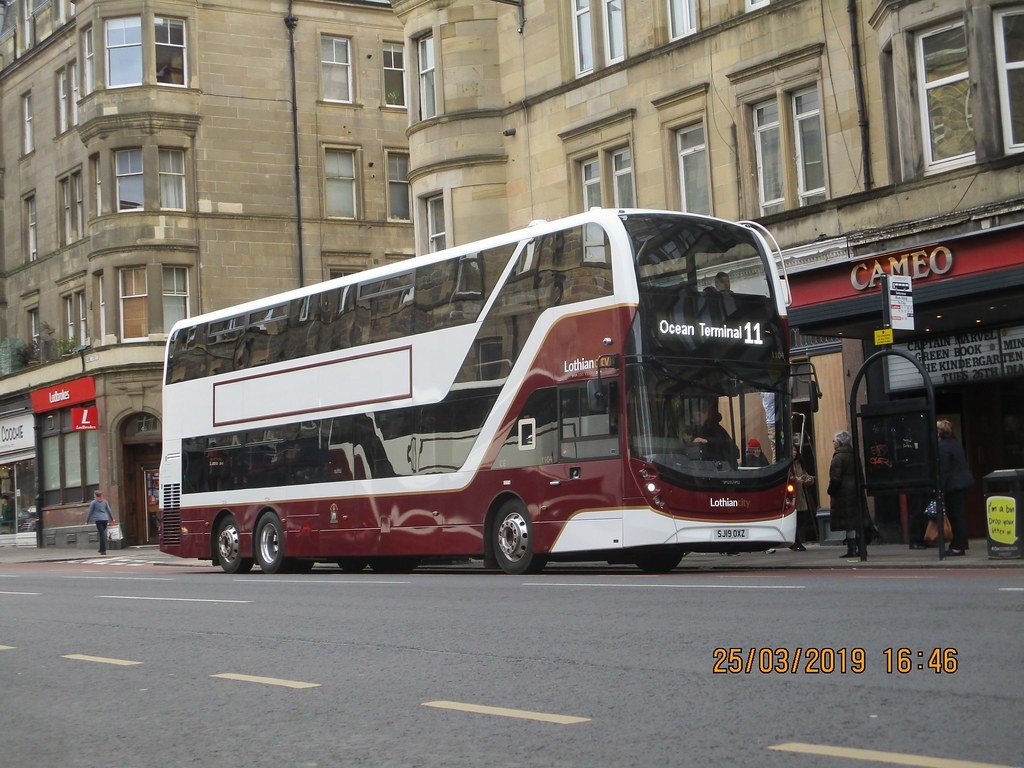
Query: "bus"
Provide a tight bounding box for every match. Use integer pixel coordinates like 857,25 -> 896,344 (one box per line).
157,204 -> 824,577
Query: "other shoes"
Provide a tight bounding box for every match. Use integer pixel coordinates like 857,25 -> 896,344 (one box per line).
945,547 -> 966,556
100,552 -> 106,555
789,544 -> 806,551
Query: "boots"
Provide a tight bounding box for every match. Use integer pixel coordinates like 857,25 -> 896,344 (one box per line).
839,537 -> 867,558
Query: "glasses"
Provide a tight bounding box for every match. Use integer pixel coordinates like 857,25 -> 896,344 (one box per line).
833,440 -> 836,443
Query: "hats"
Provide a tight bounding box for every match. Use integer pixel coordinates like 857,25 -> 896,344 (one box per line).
835,432 -> 851,447
748,439 -> 761,448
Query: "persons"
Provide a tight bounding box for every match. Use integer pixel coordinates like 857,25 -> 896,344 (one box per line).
85,490 -> 116,555
715,272 -> 730,294
827,430 -> 879,559
740,439 -> 769,467
788,444 -> 814,551
937,420 -> 980,557
691,400 -> 738,471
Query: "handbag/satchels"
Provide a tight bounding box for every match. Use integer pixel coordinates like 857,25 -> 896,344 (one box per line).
107,521 -> 123,542
943,462 -> 974,495
924,489 -> 953,543
